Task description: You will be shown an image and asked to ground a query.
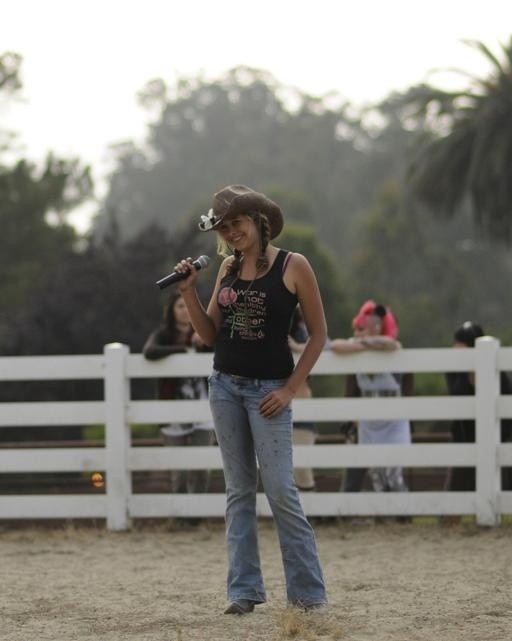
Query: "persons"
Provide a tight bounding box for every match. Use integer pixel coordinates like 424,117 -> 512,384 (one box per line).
327,304 -> 415,526
340,374 -> 369,491
174,185 -> 327,616
283,304 -> 314,490
143,292 -> 215,530
445,322 -> 512,491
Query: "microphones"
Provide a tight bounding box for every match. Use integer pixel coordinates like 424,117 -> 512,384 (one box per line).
155,255 -> 209,291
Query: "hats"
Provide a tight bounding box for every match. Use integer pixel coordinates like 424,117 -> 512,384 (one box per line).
199,185 -> 282,240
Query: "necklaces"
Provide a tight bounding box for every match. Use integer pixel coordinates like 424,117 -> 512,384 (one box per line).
226,260 -> 260,326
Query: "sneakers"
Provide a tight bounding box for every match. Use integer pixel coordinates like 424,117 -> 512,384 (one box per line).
305,604 -> 327,613
224,598 -> 254,613
